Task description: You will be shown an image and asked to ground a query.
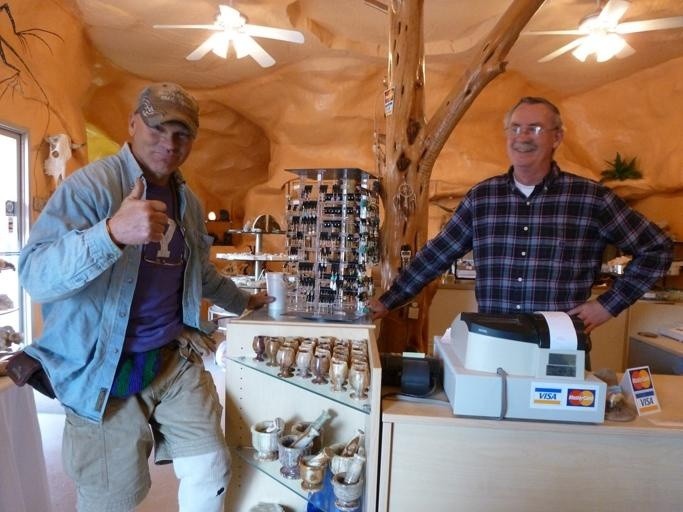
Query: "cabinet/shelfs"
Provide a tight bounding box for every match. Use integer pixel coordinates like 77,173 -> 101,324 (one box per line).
282,168 -> 379,317
216,324 -> 383,512
215,227 -> 299,295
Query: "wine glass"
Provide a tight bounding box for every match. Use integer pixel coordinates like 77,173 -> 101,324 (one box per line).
252,336 -> 370,401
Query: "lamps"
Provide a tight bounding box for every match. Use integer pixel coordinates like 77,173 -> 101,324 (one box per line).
207,5 -> 259,60
571,13 -> 628,64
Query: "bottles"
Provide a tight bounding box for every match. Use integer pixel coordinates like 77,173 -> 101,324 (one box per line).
264,270 -> 285,312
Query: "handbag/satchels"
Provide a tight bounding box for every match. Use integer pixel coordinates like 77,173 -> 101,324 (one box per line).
4,352 -> 56,398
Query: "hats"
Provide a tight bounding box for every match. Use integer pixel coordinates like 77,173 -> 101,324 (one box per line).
133,82 -> 199,138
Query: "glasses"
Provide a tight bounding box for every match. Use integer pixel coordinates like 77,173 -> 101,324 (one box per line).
502,126 -> 559,137
283,180 -> 379,306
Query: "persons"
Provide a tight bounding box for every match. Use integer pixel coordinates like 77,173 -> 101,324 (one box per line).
18,80 -> 275,511
363,96 -> 676,371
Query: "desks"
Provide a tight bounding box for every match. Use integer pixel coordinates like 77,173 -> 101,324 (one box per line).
378,371 -> 683,512
0,369 -> 54,511
626,334 -> 682,375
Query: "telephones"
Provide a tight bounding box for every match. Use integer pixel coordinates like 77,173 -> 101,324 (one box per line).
401,245 -> 412,271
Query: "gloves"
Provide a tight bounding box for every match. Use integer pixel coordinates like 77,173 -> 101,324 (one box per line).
176,330 -> 216,360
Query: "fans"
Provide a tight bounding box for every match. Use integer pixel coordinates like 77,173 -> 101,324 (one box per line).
520,0 -> 682,65
150,2 -> 305,70
